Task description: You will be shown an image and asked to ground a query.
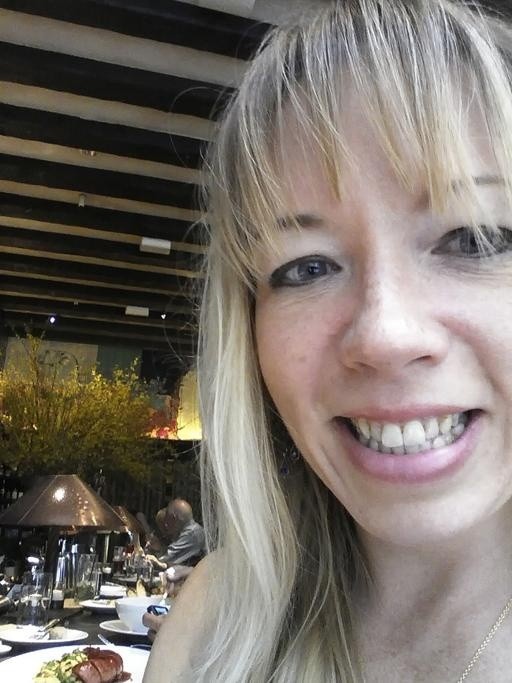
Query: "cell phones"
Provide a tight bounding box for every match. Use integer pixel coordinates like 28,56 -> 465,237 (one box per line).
146,605 -> 168,616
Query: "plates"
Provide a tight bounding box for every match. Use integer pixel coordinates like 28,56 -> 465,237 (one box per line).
0,542 -> 175,683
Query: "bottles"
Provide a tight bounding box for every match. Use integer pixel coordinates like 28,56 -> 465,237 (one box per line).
87,471 -> 107,494
0,464 -> 25,515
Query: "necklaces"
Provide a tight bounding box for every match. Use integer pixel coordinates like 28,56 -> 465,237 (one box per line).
348,593 -> 510,682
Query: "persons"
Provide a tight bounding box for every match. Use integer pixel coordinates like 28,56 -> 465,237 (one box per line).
137,0 -> 512,683
144,497 -> 206,570
146,507 -> 179,551
142,607 -> 167,643
